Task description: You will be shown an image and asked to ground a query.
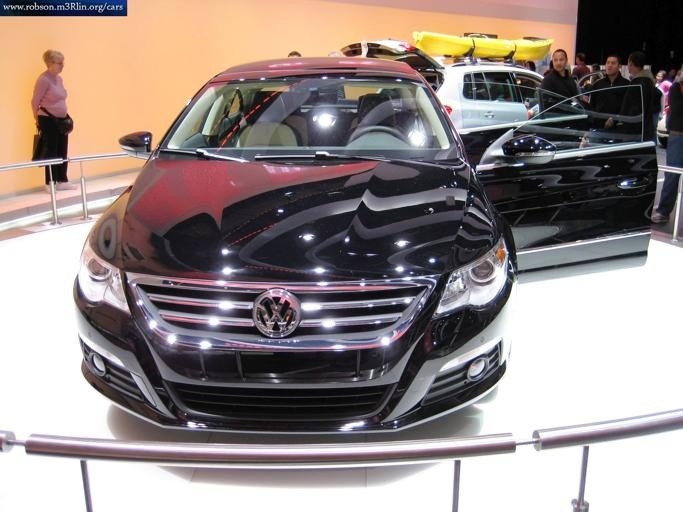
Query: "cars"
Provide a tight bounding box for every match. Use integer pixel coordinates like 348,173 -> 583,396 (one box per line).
71,37 -> 660,440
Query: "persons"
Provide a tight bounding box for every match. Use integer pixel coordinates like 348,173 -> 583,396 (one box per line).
538,49 -> 581,152
29,49 -> 77,196
519,53 -> 682,225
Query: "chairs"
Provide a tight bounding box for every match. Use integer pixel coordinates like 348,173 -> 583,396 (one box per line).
229,88 -> 420,152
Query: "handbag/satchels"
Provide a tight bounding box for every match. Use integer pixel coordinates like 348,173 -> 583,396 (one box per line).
54,115 -> 73,136
32,134 -> 41,160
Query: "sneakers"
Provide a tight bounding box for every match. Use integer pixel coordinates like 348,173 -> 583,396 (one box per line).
45,184 -> 56,194
56,181 -> 76,189
652,207 -> 666,223
